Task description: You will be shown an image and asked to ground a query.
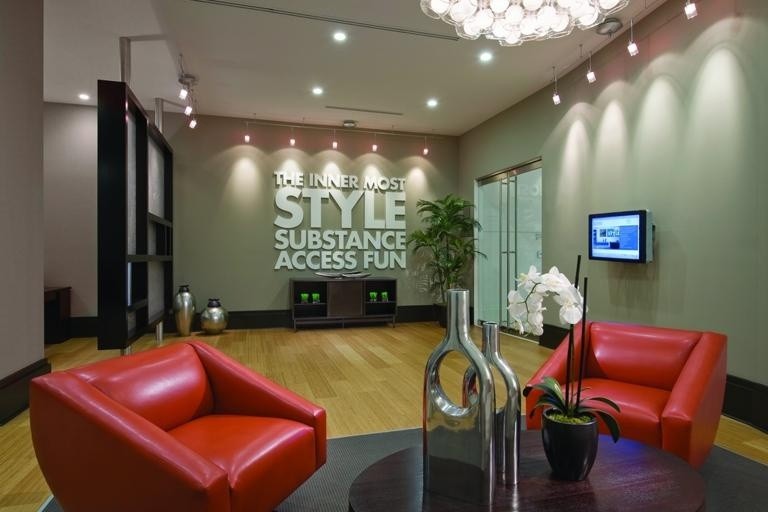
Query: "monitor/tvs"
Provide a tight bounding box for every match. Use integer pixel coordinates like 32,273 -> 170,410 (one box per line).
588,208 -> 654,264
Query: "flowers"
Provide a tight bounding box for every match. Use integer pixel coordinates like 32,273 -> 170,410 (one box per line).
509,266 -> 621,444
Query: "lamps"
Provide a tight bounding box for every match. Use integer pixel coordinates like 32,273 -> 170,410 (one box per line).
178,54 -> 197,129
420,0 -> 628,46
551,0 -> 698,106
243,121 -> 429,156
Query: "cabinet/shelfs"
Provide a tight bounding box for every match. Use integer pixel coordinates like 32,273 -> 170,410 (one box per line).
289,277 -> 398,332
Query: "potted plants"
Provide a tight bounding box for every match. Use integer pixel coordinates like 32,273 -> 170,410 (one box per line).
406,193 -> 487,328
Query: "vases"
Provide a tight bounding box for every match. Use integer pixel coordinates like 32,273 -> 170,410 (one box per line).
542,408 -> 599,482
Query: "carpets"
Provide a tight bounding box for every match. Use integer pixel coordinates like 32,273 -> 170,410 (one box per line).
44,316 -> 98,344
39,427 -> 768,512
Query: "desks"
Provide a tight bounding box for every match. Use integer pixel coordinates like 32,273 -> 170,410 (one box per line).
44,286 -> 72,323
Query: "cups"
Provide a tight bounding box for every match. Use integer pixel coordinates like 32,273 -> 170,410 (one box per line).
381,292 -> 388,303
369,292 -> 378,302
300,294 -> 309,304
313,293 -> 321,303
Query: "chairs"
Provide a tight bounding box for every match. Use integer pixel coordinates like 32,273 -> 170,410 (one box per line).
29,339 -> 327,511
523,320 -> 727,469
